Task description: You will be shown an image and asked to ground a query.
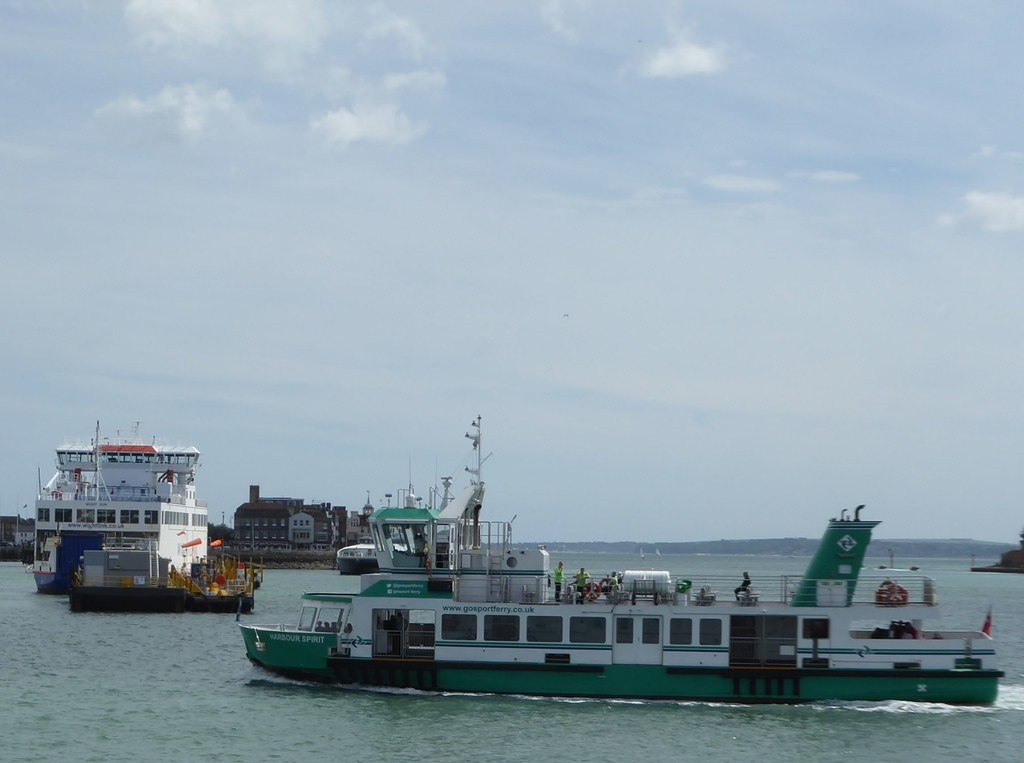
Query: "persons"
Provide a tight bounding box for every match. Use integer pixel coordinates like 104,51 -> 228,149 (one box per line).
734,572 -> 751,601
314,621 -> 352,633
554,561 -> 565,601
573,568 -> 591,604
593,572 -> 624,593
414,535 -> 426,555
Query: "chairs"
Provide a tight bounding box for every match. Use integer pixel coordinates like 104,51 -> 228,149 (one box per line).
414,539 -> 428,557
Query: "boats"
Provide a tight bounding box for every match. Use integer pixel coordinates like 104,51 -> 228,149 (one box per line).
234,413 -> 1006,707
68,532 -> 256,615
336,542 -> 410,575
31,419 -> 209,595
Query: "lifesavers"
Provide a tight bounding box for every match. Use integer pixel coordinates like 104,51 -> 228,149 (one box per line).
581,581 -> 600,602
597,577 -> 614,594
215,574 -> 225,585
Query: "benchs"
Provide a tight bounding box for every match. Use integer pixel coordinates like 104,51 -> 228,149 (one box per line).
737,584 -> 761,606
606,584 -> 629,603
692,584 -> 716,606
560,585 -> 582,605
